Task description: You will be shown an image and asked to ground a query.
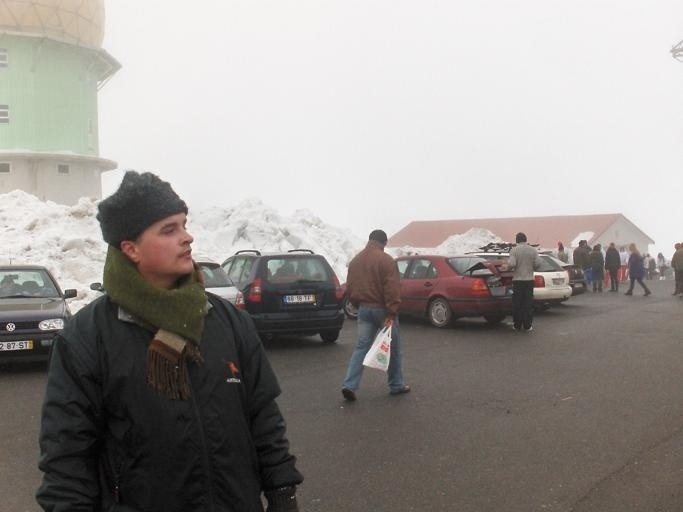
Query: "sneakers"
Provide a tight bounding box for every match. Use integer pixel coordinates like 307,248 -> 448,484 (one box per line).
390,385 -> 410,396
343,387 -> 356,401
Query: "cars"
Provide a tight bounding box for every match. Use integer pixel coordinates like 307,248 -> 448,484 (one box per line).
1,264 -> 77,369
89,255 -> 245,312
333,255 -> 512,327
537,253 -> 585,302
467,252 -> 571,309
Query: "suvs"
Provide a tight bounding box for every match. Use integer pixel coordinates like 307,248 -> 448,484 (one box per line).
221,249 -> 344,344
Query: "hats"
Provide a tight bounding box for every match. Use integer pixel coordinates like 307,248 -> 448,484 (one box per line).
96,170 -> 188,251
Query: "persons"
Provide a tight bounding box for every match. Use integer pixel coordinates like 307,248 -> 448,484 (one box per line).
504,232 -> 541,334
658,253 -> 667,281
36,169 -> 304,512
342,229 -> 412,403
670,242 -> 683,298
555,240 -> 656,296
